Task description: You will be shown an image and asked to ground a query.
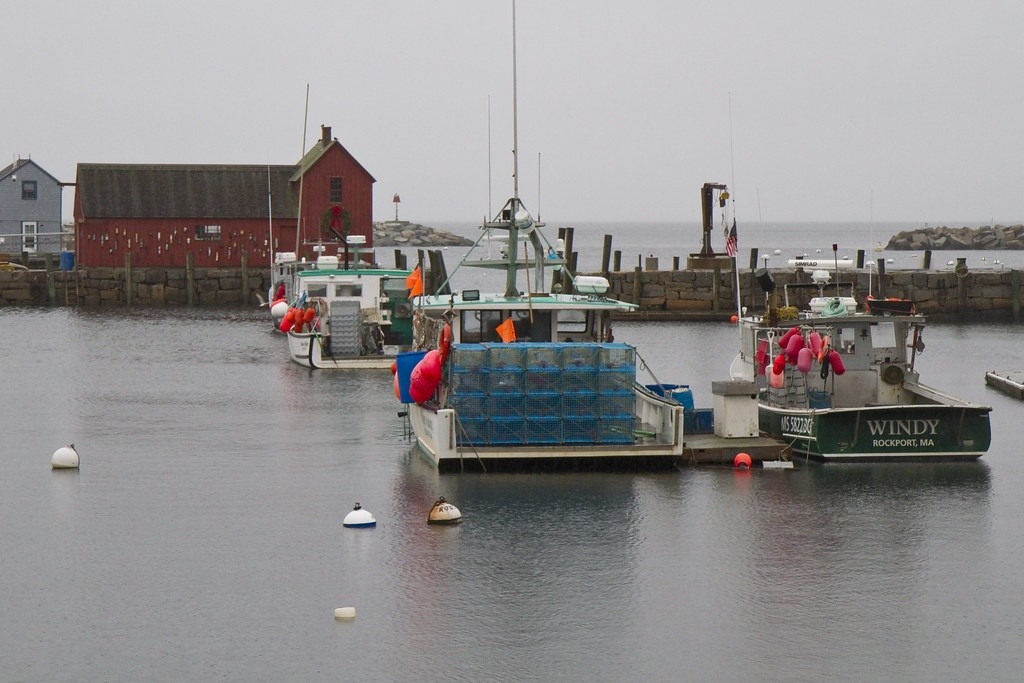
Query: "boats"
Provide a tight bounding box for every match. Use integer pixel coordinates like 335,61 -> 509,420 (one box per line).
396,5 -> 687,474
808,295 -> 857,317
265,84 -> 420,370
722,90 -> 993,466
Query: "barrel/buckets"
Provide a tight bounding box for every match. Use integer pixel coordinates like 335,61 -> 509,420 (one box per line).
665,389 -> 713,433
809,391 -> 832,409
396,350 -> 434,403
645,385 -> 689,398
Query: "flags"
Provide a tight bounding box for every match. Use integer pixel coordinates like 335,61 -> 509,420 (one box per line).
495,318 -> 516,342
725,223 -> 738,257
406,266 -> 423,299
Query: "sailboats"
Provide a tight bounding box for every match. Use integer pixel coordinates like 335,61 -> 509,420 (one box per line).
862,183 -> 914,317
